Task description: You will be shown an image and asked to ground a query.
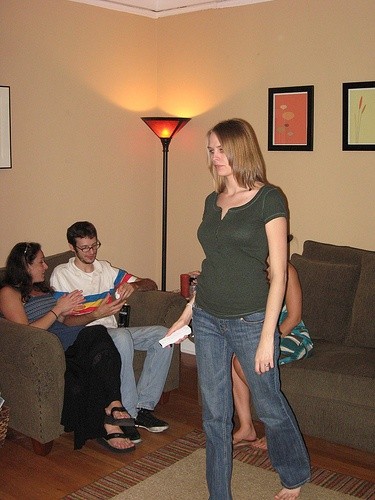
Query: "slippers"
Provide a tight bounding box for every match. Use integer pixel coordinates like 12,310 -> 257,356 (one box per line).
232,435 -> 259,449
99,433 -> 136,453
106,406 -> 136,428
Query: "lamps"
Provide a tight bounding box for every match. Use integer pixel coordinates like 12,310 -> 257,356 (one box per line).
140,114 -> 193,291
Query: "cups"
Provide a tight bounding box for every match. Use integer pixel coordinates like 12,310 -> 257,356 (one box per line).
118,304 -> 130,327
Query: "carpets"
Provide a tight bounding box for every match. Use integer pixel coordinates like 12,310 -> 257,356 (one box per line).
62,429 -> 375,500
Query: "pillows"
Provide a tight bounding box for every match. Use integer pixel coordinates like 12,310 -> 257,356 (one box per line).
289,251 -> 360,346
341,251 -> 375,350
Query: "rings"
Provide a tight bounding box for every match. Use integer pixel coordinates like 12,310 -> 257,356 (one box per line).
265,364 -> 269,367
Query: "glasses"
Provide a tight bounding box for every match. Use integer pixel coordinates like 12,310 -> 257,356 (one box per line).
75,240 -> 101,252
24,240 -> 31,255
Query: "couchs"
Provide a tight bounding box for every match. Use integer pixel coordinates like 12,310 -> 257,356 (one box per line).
0,249 -> 191,456
179,239 -> 375,451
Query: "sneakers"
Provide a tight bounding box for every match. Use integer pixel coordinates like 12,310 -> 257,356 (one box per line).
121,426 -> 142,443
133,408 -> 168,432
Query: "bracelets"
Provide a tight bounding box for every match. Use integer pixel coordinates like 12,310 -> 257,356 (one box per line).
186,303 -> 192,307
49,309 -> 58,319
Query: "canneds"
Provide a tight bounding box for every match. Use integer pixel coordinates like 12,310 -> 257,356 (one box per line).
118,303 -> 131,328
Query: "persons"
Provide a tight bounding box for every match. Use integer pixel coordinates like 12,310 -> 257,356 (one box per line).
163,118 -> 312,500
231,234 -> 314,449
49,220 -> 174,442
0,242 -> 136,454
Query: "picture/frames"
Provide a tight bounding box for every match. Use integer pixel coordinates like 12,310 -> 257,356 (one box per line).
0,84 -> 14,170
266,83 -> 316,154
340,79 -> 375,153
